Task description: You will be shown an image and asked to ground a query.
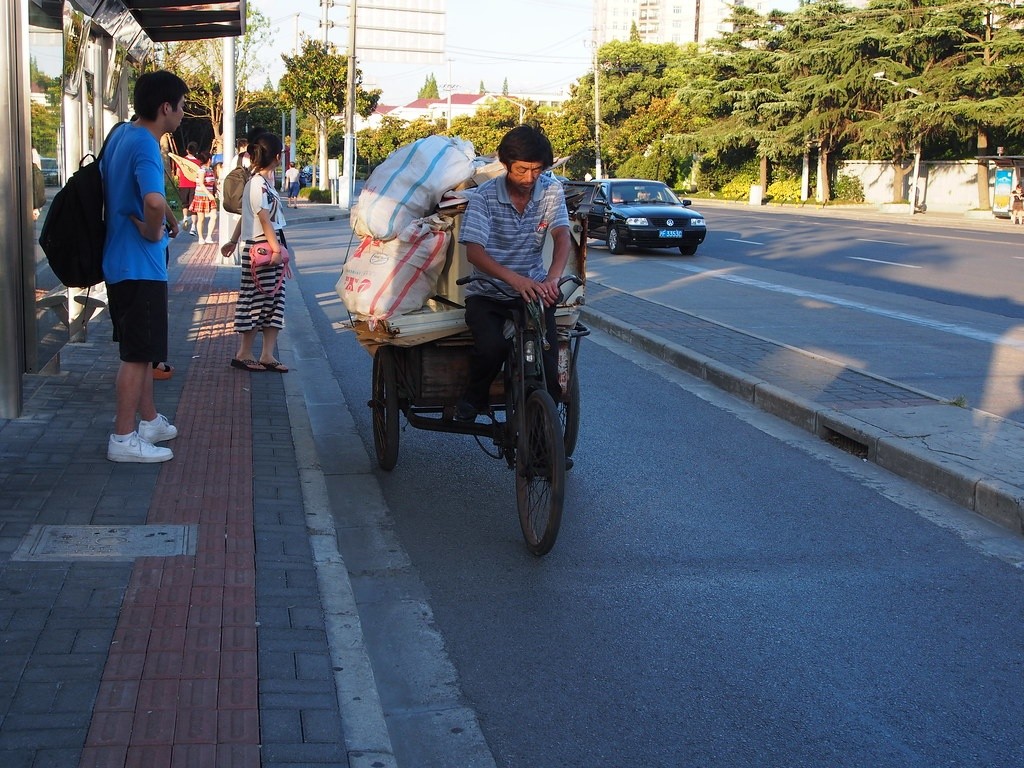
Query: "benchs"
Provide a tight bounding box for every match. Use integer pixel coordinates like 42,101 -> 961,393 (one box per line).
35,281 -> 69,328
68,280 -> 109,332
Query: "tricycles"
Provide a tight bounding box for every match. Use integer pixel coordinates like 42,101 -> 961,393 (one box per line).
368,275 -> 591,555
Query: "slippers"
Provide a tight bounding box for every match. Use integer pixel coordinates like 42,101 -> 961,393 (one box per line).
231,359 -> 266,372
258,360 -> 289,373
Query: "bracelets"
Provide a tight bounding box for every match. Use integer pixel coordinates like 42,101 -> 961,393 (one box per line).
230,240 -> 237,244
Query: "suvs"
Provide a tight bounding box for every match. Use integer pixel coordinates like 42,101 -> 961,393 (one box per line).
40,158 -> 58,186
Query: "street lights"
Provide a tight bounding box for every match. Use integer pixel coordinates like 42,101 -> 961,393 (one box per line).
484,92 -> 527,127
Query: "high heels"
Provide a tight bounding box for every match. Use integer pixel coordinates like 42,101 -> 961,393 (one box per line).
152,362 -> 175,380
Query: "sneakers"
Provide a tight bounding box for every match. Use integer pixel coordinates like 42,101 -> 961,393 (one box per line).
138,413 -> 178,443
205,237 -> 213,243
182,217 -> 188,231
107,431 -> 174,463
199,239 -> 206,244
189,229 -> 197,236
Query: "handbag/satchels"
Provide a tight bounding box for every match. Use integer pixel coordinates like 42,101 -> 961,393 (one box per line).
248,243 -> 293,296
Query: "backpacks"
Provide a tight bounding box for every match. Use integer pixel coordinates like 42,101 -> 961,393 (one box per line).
223,151 -> 255,215
39,121 -> 127,289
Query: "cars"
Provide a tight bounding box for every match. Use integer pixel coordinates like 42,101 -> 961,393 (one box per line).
583,179 -> 706,255
303,165 -> 319,182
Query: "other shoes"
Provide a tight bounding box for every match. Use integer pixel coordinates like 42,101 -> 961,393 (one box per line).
454,397 -> 490,418
566,457 -> 573,470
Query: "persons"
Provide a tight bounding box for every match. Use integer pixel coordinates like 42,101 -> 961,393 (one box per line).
99,70 -> 190,462
1010,184 -> 1024,225
220,133 -> 291,373
633,188 -> 660,201
172,133 -> 276,236
187,151 -> 219,246
284,162 -> 301,209
584,170 -> 594,182
32,141 -> 46,220
454,125 -> 575,472
